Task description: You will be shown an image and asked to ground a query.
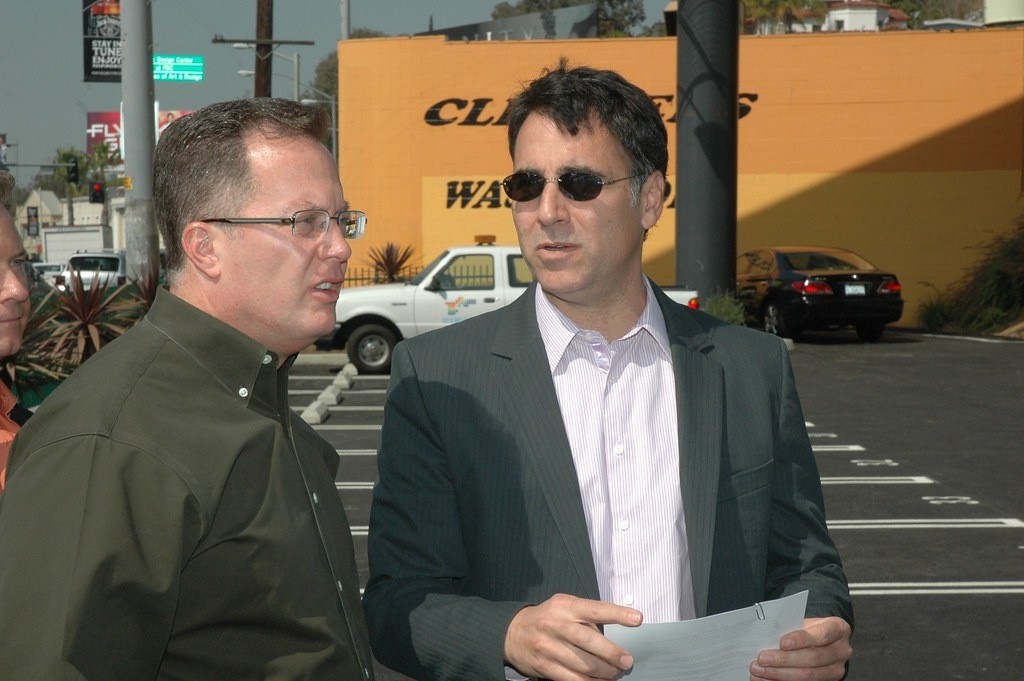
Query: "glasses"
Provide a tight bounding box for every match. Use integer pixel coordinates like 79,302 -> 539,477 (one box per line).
203,208 -> 368,239
499,169 -> 649,202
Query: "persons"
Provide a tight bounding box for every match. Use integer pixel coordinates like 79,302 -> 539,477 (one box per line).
1,98 -> 376,681
1,167 -> 31,503
359,56 -> 858,681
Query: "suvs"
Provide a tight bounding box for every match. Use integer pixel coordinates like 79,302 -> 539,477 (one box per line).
53,248 -> 125,293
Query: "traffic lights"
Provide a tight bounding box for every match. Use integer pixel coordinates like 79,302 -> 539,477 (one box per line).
87,178 -> 106,203
26,206 -> 40,237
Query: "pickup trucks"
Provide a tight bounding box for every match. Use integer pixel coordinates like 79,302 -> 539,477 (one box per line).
312,245 -> 701,376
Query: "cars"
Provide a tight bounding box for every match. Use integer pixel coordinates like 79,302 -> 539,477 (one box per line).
736,244 -> 905,343
30,261 -> 67,288
